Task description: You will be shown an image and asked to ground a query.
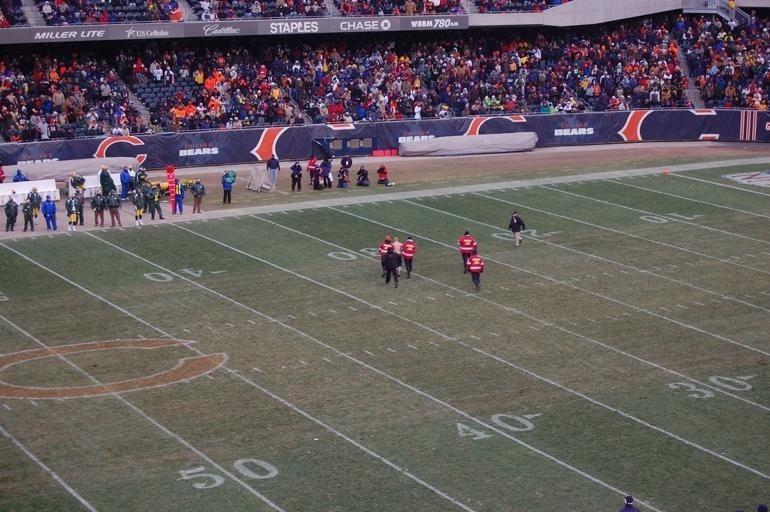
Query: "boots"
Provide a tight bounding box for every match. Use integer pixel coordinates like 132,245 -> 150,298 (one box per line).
67,225 -> 72,231
136,220 -> 139,226
72,226 -> 76,232
139,219 -> 143,225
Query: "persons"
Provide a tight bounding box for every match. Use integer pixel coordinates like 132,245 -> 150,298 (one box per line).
508,211 -> 526,247
457,230 -> 477,273
400,236 -> 417,279
618,494 -> 638,512
1,0 -> 770,147
392,236 -> 403,276
381,248 -> 402,288
465,251 -> 485,292
0,152 -> 389,233
379,235 -> 392,276
